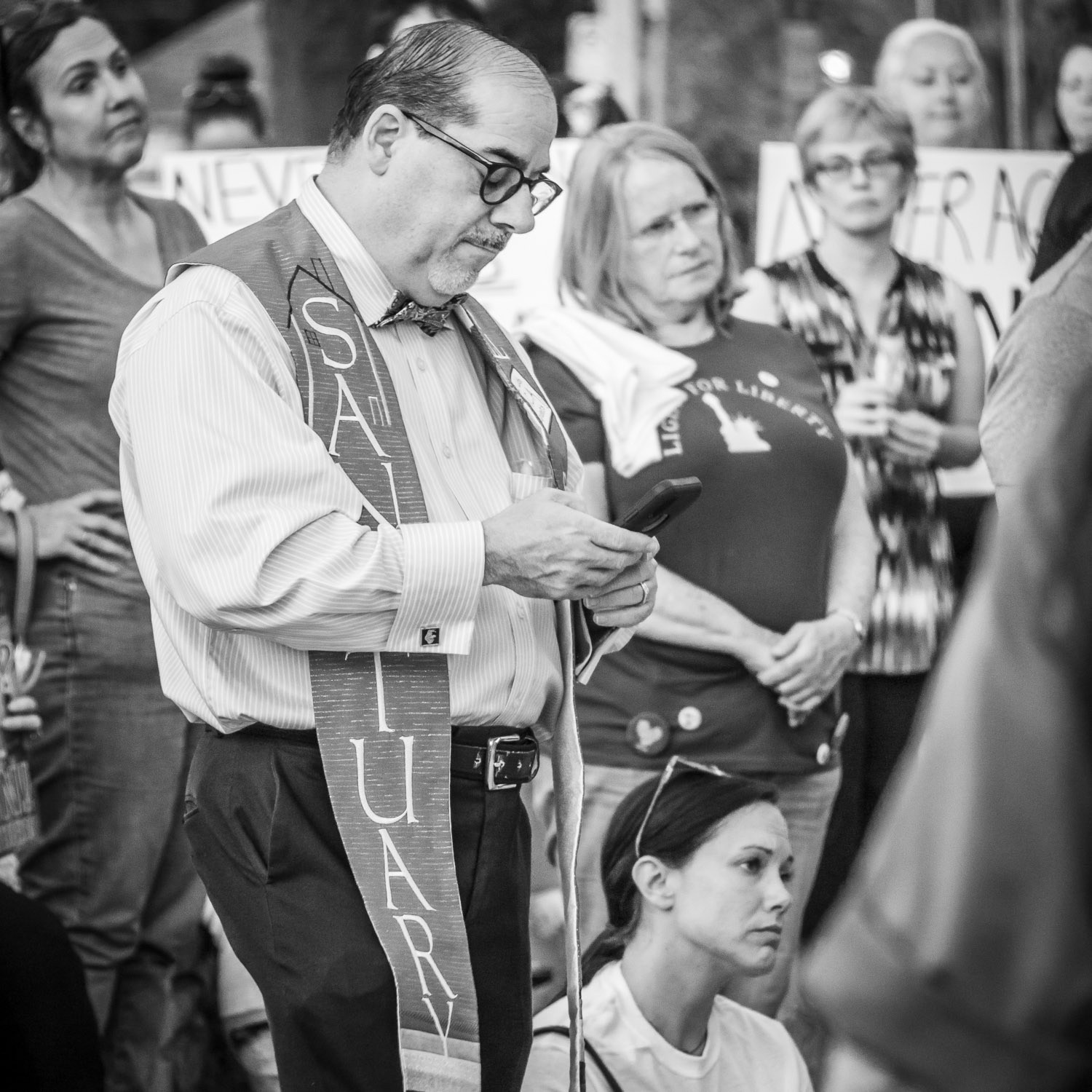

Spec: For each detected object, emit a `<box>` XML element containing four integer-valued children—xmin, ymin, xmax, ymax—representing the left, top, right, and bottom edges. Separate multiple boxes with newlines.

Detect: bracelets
<box><xmin>826</xmin><ymin>604</ymin><xmax>866</xmax><ymax>643</ymax></box>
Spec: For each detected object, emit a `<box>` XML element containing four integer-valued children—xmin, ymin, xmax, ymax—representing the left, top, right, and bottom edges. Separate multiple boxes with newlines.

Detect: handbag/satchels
<box><xmin>0</xmin><ymin>504</ymin><xmax>52</xmax><ymax>859</ymax></box>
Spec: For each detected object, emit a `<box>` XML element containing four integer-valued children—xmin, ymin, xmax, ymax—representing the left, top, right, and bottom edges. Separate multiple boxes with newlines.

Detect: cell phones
<box><xmin>612</xmin><ymin>477</ymin><xmax>704</xmax><ymax>538</ymax></box>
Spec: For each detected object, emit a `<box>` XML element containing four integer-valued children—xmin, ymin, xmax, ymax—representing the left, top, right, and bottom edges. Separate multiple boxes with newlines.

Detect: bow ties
<box><xmin>368</xmin><ymin>290</ymin><xmax>470</xmax><ymax>338</ymax></box>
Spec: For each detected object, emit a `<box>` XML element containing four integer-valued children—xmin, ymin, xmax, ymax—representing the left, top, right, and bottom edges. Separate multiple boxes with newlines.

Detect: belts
<box><xmin>202</xmin><ymin>721</ymin><xmax>542</xmax><ymax>793</ymax></box>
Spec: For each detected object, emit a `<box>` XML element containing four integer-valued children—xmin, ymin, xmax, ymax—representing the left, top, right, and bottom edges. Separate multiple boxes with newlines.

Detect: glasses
<box><xmin>395</xmin><ymin>107</ymin><xmax>565</xmax><ymax>219</ymax></box>
<box><xmin>633</xmin><ymin>753</ymin><xmax>736</xmax><ymax>862</ymax></box>
<box><xmin>802</xmin><ymin>149</ymin><xmax>906</xmax><ymax>181</ymax></box>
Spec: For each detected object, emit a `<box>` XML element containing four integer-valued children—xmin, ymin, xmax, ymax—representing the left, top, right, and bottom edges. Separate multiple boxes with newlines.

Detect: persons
<box><xmin>795</xmin><ymin>16</ymin><xmax>1092</xmax><ymax>1092</ymax></box>
<box><xmin>0</xmin><ymin>0</ymin><xmax>220</xmax><ymax>1092</ymax></box>
<box><xmin>519</xmin><ymin>770</ymin><xmax>816</xmax><ymax>1092</ymax></box>
<box><xmin>512</xmin><ymin>119</ymin><xmax>881</xmax><ymax>1019</ymax></box>
<box><xmin>177</xmin><ymin>55</ymin><xmax>265</xmax><ymax>152</ymax></box>
<box><xmin>727</xmin><ymin>84</ymin><xmax>987</xmax><ymax>949</ymax></box>
<box><xmin>105</xmin><ymin>18</ymin><xmax>661</xmax><ymax>1091</ymax></box>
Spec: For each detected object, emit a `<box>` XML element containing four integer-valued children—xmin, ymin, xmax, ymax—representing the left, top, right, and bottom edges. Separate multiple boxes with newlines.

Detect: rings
<box><xmin>634</xmin><ymin>582</ymin><xmax>649</xmax><ymax>606</ymax></box>
